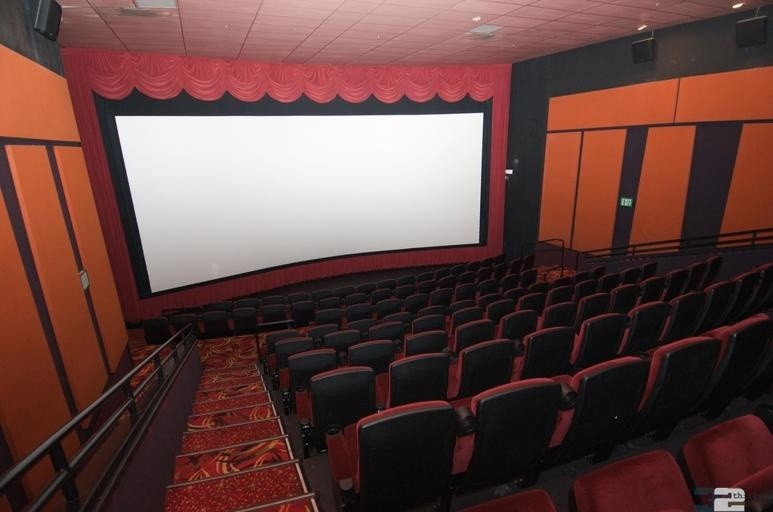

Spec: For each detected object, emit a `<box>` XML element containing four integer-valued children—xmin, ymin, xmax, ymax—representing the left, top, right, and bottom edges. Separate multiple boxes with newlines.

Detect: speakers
<box><xmin>33</xmin><ymin>0</ymin><xmax>62</xmax><ymax>41</ymax></box>
<box><xmin>736</xmin><ymin>16</ymin><xmax>766</xmax><ymax>47</ymax></box>
<box><xmin>631</xmin><ymin>38</ymin><xmax>655</xmax><ymax>63</ymax></box>
